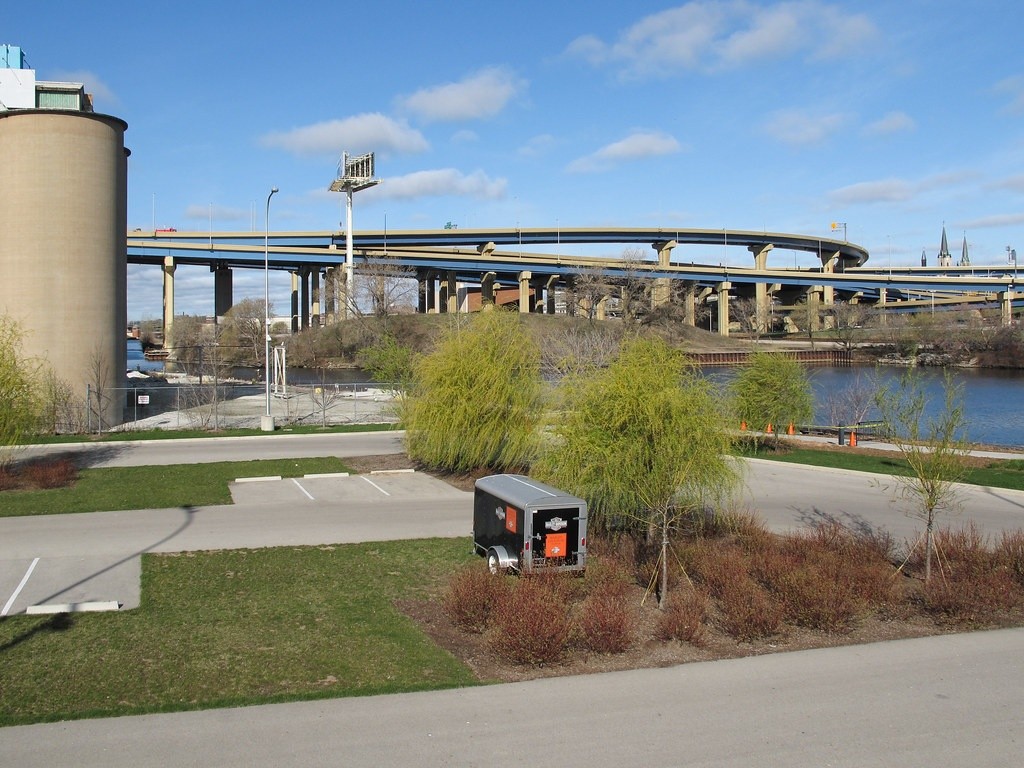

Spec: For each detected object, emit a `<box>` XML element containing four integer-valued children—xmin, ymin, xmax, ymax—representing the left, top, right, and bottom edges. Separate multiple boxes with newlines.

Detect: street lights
<box><xmin>770</xmin><ymin>301</ymin><xmax>775</xmax><ymax>333</ymax></box>
<box><xmin>887</xmin><ymin>234</ymin><xmax>891</xmax><ymax>276</ymax></box>
<box><xmin>555</xmin><ymin>219</ymin><xmax>560</xmax><ymax>260</ymax></box>
<box><xmin>722</xmin><ymin>227</ymin><xmax>728</xmax><ymax>269</ymax></box>
<box><xmin>383</xmin><ymin>209</ymin><xmax>388</xmax><ymax>256</ymax></box>
<box><xmin>930</xmin><ymin>290</ymin><xmax>936</xmax><ymax>323</ymax></box>
<box><xmin>708</xmin><ymin>305</ymin><xmax>713</xmax><ymax>332</ymax></box>
<box><xmin>265</xmin><ymin>187</ymin><xmax>279</xmax><ymax>417</ymax></box>
<box><xmin>516</xmin><ymin>221</ymin><xmax>522</xmax><ymax>258</ymax></box>
<box><xmin>882</xmin><ymin>291</ymin><xmax>888</xmax><ymax>305</ymax></box>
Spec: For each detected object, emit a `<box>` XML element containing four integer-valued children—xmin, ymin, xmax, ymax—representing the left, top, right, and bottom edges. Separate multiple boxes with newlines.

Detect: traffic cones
<box><xmin>740</xmin><ymin>421</ymin><xmax>747</xmax><ymax>431</ymax></box>
<box><xmin>848</xmin><ymin>431</ymin><xmax>856</xmax><ymax>447</ymax></box>
<box><xmin>766</xmin><ymin>422</ymin><xmax>773</xmax><ymax>434</ymax></box>
<box><xmin>787</xmin><ymin>422</ymin><xmax>795</xmax><ymax>435</ymax></box>
